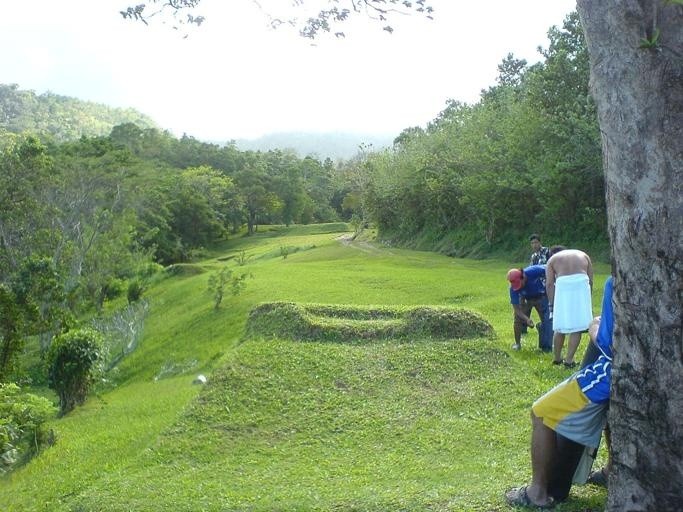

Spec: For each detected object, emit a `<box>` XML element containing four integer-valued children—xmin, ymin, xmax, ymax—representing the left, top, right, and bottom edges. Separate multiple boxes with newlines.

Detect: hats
<box><xmin>507</xmin><ymin>269</ymin><xmax>522</xmax><ymax>291</ymax></box>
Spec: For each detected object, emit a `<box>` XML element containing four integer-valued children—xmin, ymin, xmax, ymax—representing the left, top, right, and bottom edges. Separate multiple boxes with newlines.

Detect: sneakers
<box><xmin>562</xmin><ymin>360</ymin><xmax>580</xmax><ymax>368</ymax></box>
<box><xmin>552</xmin><ymin>360</ymin><xmax>563</xmax><ymax>365</ymax></box>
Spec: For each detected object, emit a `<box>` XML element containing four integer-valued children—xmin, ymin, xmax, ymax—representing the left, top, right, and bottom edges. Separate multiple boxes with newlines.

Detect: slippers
<box><xmin>587</xmin><ymin>467</ymin><xmax>610</xmax><ymax>489</ymax></box>
<box><xmin>506</xmin><ymin>485</ymin><xmax>555</xmax><ymax>509</ymax></box>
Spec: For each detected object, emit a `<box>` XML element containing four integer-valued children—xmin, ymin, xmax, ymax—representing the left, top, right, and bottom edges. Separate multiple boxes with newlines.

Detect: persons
<box><xmin>502</xmin><ymin>267</ymin><xmax>615</xmax><ymax>510</ymax></box>
<box><xmin>506</xmin><ymin>265</ymin><xmax>548</xmax><ymax>350</ymax></box>
<box><xmin>527</xmin><ymin>234</ymin><xmax>550</xmax><ymax>267</ymax></box>
<box><xmin>544</xmin><ymin>245</ymin><xmax>594</xmax><ymax>368</ymax></box>
